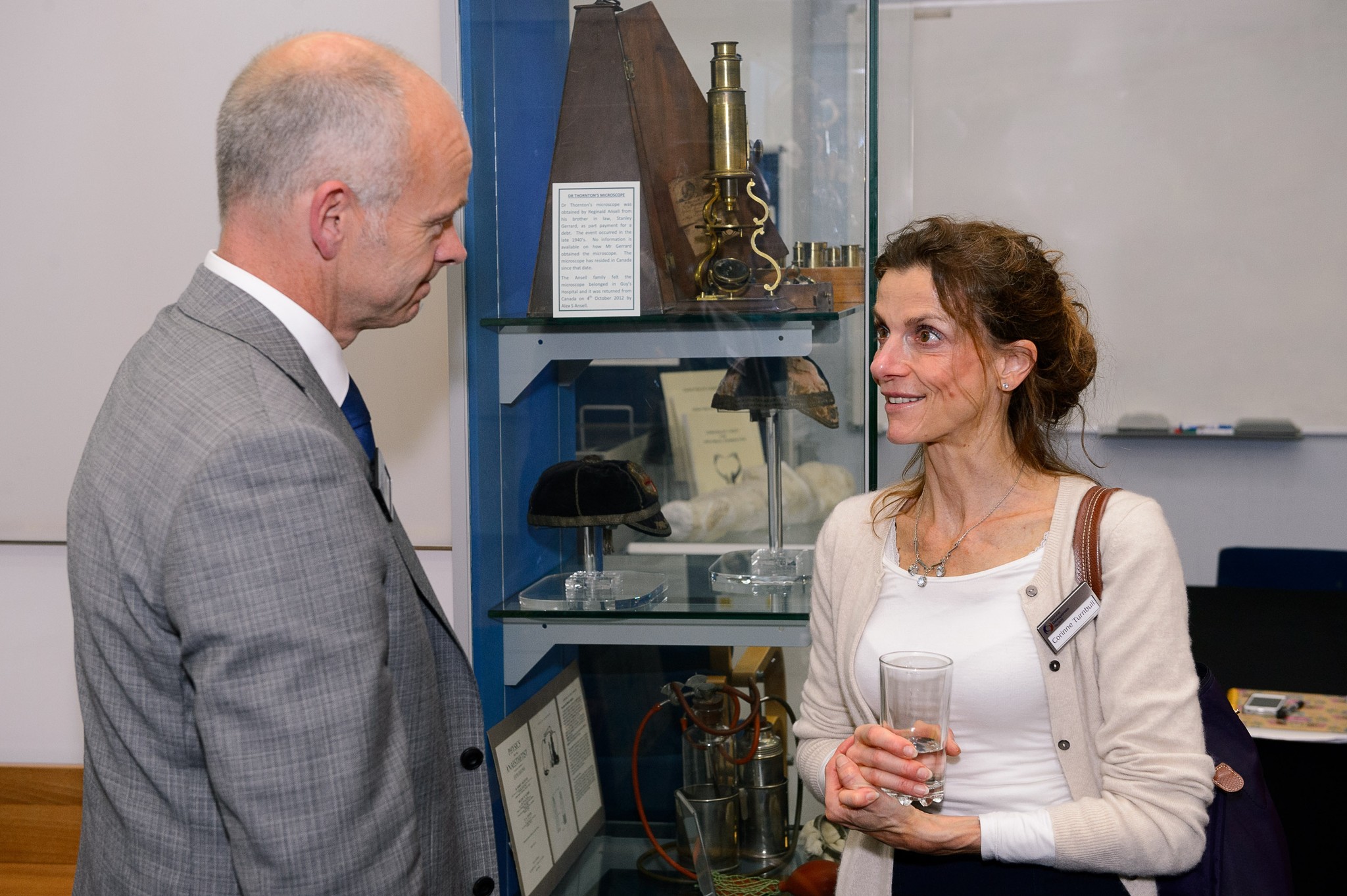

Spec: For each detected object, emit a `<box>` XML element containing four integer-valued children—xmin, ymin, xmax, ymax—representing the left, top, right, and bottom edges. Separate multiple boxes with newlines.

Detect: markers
<box><xmin>1176</xmin><ymin>423</ymin><xmax>1234</xmax><ymax>436</ymax></box>
<box><xmin>1276</xmin><ymin>700</ymin><xmax>1305</xmax><ymax>719</ymax></box>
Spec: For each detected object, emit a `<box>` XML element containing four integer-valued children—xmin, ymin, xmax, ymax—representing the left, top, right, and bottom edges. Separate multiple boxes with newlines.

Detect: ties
<box><xmin>344</xmin><ymin>374</ymin><xmax>374</xmax><ymax>470</ymax></box>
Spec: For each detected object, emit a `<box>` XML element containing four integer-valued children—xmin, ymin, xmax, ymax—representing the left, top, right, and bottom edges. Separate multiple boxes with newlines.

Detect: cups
<box><xmin>879</xmin><ymin>652</ymin><xmax>953</xmax><ymax>805</ymax></box>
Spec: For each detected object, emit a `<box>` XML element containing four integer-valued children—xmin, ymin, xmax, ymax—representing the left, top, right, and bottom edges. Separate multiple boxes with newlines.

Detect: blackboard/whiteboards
<box><xmin>866</xmin><ymin>1</ymin><xmax>1347</xmax><ymax>438</ymax></box>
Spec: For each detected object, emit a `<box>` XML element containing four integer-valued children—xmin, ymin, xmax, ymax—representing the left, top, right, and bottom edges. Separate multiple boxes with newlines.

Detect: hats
<box><xmin>713</xmin><ymin>354</ymin><xmax>840</xmax><ymax>429</ymax></box>
<box><xmin>528</xmin><ymin>454</ymin><xmax>672</xmax><ymax>536</ymax></box>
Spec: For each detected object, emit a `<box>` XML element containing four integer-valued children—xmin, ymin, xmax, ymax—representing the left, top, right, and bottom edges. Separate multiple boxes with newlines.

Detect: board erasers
<box><xmin>1233</xmin><ymin>418</ymin><xmax>1303</xmax><ymax>436</ymax></box>
<box><xmin>1117</xmin><ymin>413</ymin><xmax>1170</xmax><ymax>435</ymax></box>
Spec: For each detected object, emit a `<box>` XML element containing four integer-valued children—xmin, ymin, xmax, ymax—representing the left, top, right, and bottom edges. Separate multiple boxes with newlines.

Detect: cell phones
<box><xmin>1243</xmin><ymin>692</ymin><xmax>1286</xmax><ymax>716</ymax></box>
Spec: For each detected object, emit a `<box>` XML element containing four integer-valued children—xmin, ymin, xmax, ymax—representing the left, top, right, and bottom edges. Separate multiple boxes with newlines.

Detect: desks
<box><xmin>1228</xmin><ymin>688</ymin><xmax>1347</xmax><ymax>743</ymax></box>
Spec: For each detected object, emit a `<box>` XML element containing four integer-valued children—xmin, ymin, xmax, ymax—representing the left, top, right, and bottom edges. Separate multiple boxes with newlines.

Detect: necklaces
<box><xmin>904</xmin><ymin>453</ymin><xmax>1026</xmax><ymax>589</ymax></box>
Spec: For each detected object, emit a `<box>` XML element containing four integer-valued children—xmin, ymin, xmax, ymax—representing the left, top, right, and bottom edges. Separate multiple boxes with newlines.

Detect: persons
<box><xmin>66</xmin><ymin>32</ymin><xmax>500</xmax><ymax>896</ymax></box>
<box><xmin>790</xmin><ymin>217</ymin><xmax>1215</xmax><ymax>896</ymax></box>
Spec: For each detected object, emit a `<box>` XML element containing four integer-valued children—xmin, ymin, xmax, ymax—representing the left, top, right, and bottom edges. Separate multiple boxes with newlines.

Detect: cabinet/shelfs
<box><xmin>454</xmin><ymin>0</ymin><xmax>879</xmax><ymax>896</ymax></box>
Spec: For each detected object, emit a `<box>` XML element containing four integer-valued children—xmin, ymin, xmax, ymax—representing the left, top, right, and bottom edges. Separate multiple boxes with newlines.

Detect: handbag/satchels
<box><xmin>1077</xmin><ymin>483</ymin><xmax>1289</xmax><ymax>895</ymax></box>
